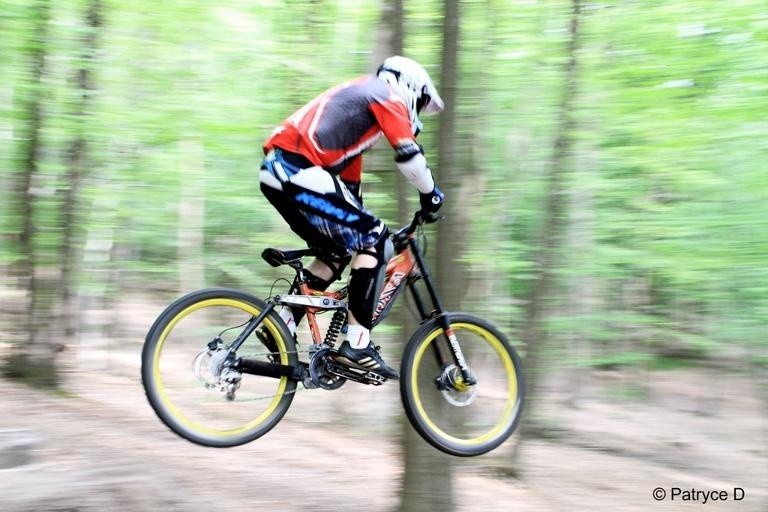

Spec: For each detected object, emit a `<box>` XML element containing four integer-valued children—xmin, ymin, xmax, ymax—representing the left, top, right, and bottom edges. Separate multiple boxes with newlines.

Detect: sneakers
<box><xmin>337</xmin><ymin>340</ymin><xmax>402</xmax><ymax>379</ymax></box>
<box><xmin>256</xmin><ymin>324</ymin><xmax>297</xmax><ymax>363</ymax></box>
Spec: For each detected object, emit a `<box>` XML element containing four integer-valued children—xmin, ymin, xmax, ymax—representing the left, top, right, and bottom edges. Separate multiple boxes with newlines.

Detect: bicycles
<box><xmin>140</xmin><ymin>195</ymin><xmax>526</xmax><ymax>457</ymax></box>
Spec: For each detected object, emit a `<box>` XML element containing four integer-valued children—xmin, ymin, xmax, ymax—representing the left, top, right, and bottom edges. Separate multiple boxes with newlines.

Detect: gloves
<box><xmin>418</xmin><ymin>183</ymin><xmax>445</xmax><ymax>212</ymax></box>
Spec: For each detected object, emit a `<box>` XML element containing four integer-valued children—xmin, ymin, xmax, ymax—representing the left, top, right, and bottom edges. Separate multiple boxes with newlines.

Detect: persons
<box><xmin>259</xmin><ymin>55</ymin><xmax>449</xmax><ymax>380</ymax></box>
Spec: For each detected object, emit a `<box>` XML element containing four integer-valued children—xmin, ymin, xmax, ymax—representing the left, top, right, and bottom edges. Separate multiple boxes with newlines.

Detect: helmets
<box><xmin>377</xmin><ymin>55</ymin><xmax>445</xmax><ymax>113</ymax></box>
